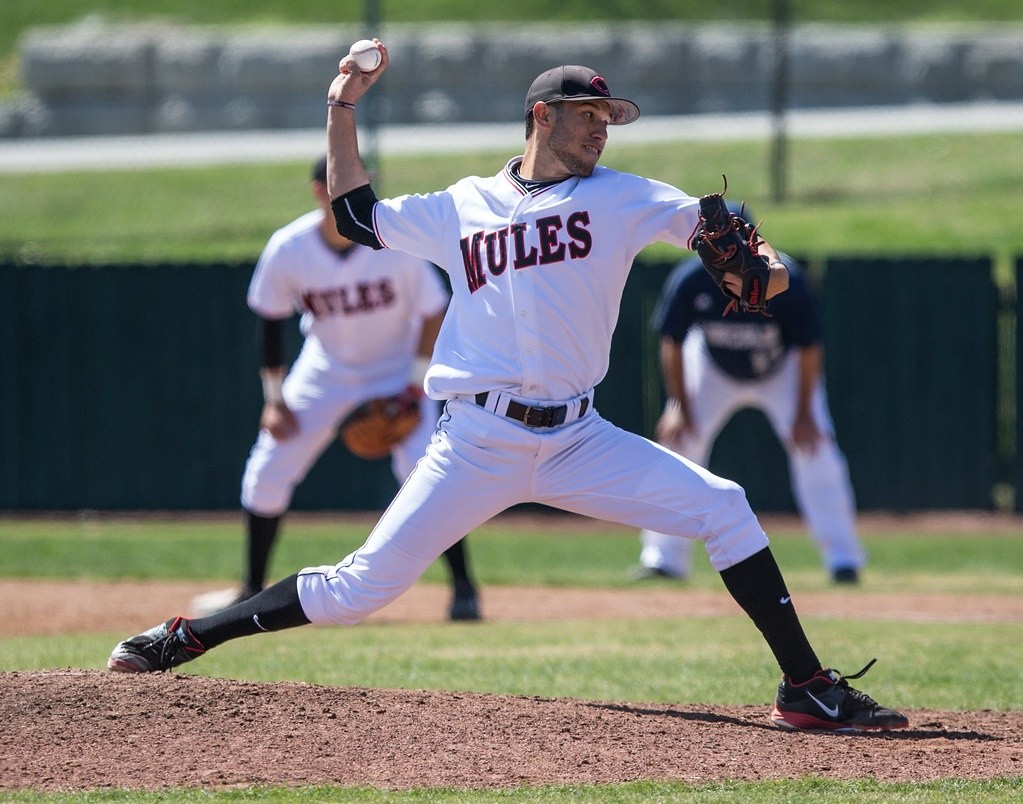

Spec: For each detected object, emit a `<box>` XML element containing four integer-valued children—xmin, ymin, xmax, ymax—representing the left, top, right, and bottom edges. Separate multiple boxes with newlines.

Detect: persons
<box><xmin>219</xmin><ymin>153</ymin><xmax>478</xmax><ymax>620</ymax></box>
<box><xmin>626</xmin><ymin>199</ymin><xmax>866</xmax><ymax>582</ymax></box>
<box><xmin>108</xmin><ymin>37</ymin><xmax>909</xmax><ymax>732</ymax></box>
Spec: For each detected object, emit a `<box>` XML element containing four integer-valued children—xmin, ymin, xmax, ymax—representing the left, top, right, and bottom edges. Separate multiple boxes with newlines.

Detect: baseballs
<box><xmin>349</xmin><ymin>39</ymin><xmax>382</xmax><ymax>73</ymax></box>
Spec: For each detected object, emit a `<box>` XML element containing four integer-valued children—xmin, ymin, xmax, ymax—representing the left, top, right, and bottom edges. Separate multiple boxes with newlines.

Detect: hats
<box><xmin>314</xmin><ymin>157</ymin><xmax>326</xmax><ymax>180</ymax></box>
<box><xmin>729</xmin><ymin>203</ymin><xmax>751</xmax><ymax>226</ymax></box>
<box><xmin>524</xmin><ymin>65</ymin><xmax>640</xmax><ymax>125</ymax></box>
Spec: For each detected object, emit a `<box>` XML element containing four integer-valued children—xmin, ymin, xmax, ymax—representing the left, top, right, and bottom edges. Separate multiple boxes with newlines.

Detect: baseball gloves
<box><xmin>343</xmin><ymin>386</ymin><xmax>422</xmax><ymax>460</ymax></box>
<box><xmin>691</xmin><ymin>192</ymin><xmax>772</xmax><ymax>311</ymax></box>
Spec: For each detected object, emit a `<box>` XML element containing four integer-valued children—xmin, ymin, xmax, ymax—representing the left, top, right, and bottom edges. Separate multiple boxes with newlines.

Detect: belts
<box><xmin>475</xmin><ymin>392</ymin><xmax>589</xmax><ymax>427</ymax></box>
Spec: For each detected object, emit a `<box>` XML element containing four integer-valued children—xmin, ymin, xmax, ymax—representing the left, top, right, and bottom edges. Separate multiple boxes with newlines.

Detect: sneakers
<box><xmin>771</xmin><ymin>658</ymin><xmax>909</xmax><ymax>731</ymax></box>
<box><xmin>108</xmin><ymin>617</ymin><xmax>206</xmax><ymax>672</ymax></box>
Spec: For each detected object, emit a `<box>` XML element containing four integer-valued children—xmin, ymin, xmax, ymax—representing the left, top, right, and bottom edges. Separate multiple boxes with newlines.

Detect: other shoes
<box><xmin>634</xmin><ymin>567</ymin><xmax>674</xmax><ymax>580</ymax></box>
<box><xmin>833</xmin><ymin>568</ymin><xmax>859</xmax><ymax>584</ymax></box>
<box><xmin>453</xmin><ymin>585</ymin><xmax>478</xmax><ymax>620</ymax></box>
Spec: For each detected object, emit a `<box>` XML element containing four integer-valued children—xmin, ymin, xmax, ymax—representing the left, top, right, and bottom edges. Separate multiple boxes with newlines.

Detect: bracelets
<box><xmin>326</xmin><ymin>100</ymin><xmax>357</xmax><ymax>112</ymax></box>
